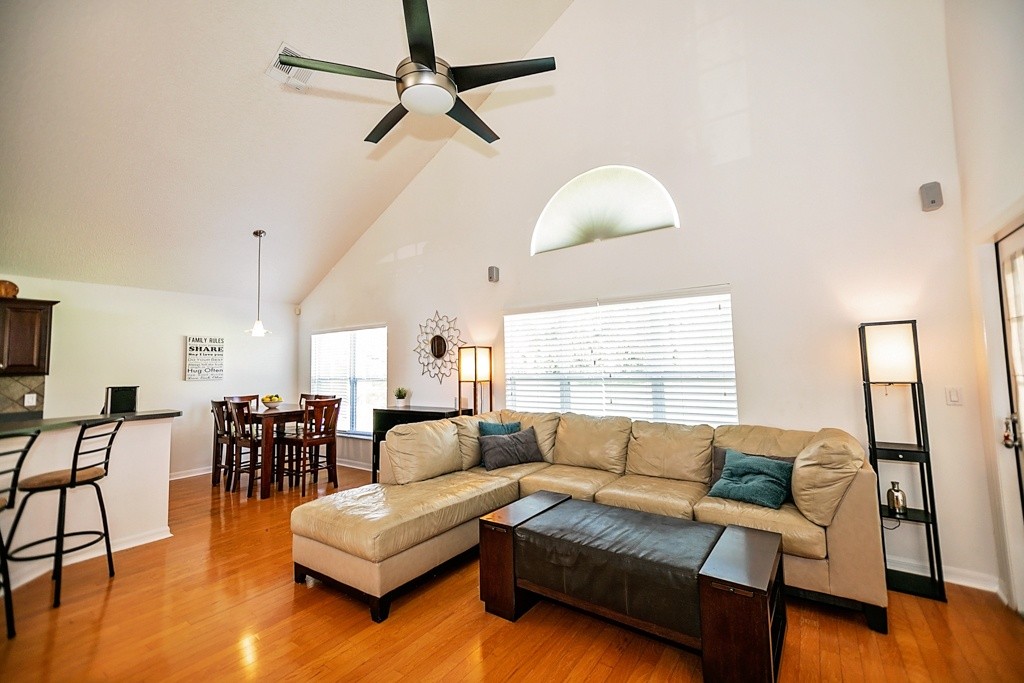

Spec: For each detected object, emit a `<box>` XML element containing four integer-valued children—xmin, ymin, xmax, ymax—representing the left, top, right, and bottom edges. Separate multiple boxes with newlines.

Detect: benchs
<box><xmin>478</xmin><ymin>489</ymin><xmax>789</xmax><ymax>683</ymax></box>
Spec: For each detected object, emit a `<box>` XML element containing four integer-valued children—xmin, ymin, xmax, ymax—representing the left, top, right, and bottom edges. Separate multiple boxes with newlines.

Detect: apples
<box><xmin>262</xmin><ymin>395</ymin><xmax>282</xmax><ymax>401</ymax></box>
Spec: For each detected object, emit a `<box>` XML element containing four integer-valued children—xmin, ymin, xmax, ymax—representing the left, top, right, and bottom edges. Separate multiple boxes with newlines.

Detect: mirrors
<box><xmin>413</xmin><ymin>309</ymin><xmax>467</xmax><ymax>384</ymax></box>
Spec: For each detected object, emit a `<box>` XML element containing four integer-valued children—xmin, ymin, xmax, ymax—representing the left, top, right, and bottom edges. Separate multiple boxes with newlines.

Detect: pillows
<box><xmin>477</xmin><ymin>426</ymin><xmax>544</xmax><ymax>472</ymax></box>
<box><xmin>478</xmin><ymin>421</ymin><xmax>520</xmax><ymax>467</ymax></box>
<box><xmin>711</xmin><ymin>445</ymin><xmax>796</xmax><ymax>487</ymax></box>
<box><xmin>707</xmin><ymin>448</ymin><xmax>793</xmax><ymax>510</ymax></box>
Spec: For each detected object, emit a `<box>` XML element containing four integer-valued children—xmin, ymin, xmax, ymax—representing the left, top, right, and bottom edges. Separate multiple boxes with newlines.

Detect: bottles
<box><xmin>887</xmin><ymin>481</ymin><xmax>907</xmax><ymax>520</ymax></box>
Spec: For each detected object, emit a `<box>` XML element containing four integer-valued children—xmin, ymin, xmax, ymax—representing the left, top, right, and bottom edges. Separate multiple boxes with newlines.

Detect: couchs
<box><xmin>290</xmin><ymin>410</ymin><xmax>889</xmax><ymax>638</ymax></box>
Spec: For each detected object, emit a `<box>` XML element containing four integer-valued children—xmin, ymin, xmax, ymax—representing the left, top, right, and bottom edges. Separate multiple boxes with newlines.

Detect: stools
<box><xmin>0</xmin><ymin>429</ymin><xmax>41</xmax><ymax>639</ymax></box>
<box><xmin>0</xmin><ymin>414</ymin><xmax>126</xmax><ymax>608</ymax></box>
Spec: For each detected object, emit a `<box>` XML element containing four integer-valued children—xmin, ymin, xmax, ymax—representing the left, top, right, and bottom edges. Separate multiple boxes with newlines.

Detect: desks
<box><xmin>211</xmin><ymin>404</ymin><xmax>338</xmax><ymax>499</ymax></box>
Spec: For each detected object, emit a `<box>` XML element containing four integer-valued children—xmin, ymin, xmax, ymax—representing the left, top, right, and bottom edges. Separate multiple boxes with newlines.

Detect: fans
<box><xmin>279</xmin><ymin>0</ymin><xmax>556</xmax><ymax>143</ymax></box>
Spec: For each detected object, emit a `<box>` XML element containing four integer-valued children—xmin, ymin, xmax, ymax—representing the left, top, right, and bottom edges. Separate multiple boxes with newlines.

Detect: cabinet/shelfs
<box><xmin>858</xmin><ymin>319</ymin><xmax>948</xmax><ymax>604</ymax></box>
<box><xmin>372</xmin><ymin>405</ymin><xmax>473</xmax><ymax>484</ymax></box>
<box><xmin>0</xmin><ymin>295</ymin><xmax>61</xmax><ymax>376</ymax></box>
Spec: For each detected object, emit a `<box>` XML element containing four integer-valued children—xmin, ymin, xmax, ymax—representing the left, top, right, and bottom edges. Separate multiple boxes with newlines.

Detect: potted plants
<box><xmin>392</xmin><ymin>385</ymin><xmax>407</xmax><ymax>406</ymax></box>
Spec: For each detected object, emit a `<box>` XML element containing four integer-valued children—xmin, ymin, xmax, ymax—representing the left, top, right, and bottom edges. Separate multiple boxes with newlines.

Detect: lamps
<box><xmin>396</xmin><ymin>72</ymin><xmax>459</xmax><ymax>117</ymax></box>
<box><xmin>458</xmin><ymin>346</ymin><xmax>492</xmax><ymax>416</ymax></box>
<box><xmin>251</xmin><ymin>229</ymin><xmax>268</xmax><ymax>337</ymax></box>
<box><xmin>864</xmin><ymin>325</ymin><xmax>918</xmax><ymax>397</ymax></box>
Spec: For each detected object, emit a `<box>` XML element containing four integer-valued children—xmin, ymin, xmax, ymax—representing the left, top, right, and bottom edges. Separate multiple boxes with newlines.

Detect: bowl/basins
<box><xmin>263</xmin><ymin>401</ymin><xmax>283</xmax><ymax>409</ymax></box>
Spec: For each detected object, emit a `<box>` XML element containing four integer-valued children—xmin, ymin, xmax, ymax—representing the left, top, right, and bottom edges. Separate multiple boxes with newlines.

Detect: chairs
<box><xmin>211</xmin><ymin>394</ymin><xmax>342</xmax><ymax>498</ymax></box>
